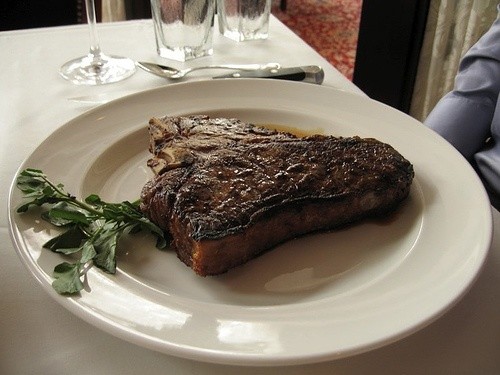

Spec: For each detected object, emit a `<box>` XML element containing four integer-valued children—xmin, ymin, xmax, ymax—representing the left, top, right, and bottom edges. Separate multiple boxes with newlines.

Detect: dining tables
<box><xmin>0</xmin><ymin>13</ymin><xmax>499</xmax><ymax>375</ymax></box>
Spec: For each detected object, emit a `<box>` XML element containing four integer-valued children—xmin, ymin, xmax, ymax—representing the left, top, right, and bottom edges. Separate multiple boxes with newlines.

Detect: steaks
<box><xmin>147</xmin><ymin>114</ymin><xmax>298</xmax><ymax>165</ymax></box>
<box><xmin>137</xmin><ymin>134</ymin><xmax>416</xmax><ymax>276</ymax></box>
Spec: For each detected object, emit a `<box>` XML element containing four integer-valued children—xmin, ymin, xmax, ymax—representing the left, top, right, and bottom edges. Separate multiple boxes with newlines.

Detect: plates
<box><xmin>7</xmin><ymin>77</ymin><xmax>495</xmax><ymax>368</ymax></box>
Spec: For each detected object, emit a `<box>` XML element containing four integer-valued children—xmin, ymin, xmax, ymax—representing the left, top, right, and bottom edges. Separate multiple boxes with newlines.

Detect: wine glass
<box><xmin>60</xmin><ymin>0</ymin><xmax>135</xmax><ymax>86</ymax></box>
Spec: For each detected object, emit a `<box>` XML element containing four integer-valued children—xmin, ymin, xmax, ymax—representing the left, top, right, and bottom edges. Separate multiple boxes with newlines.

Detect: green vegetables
<box><xmin>13</xmin><ymin>168</ymin><xmax>173</xmax><ymax>294</ymax></box>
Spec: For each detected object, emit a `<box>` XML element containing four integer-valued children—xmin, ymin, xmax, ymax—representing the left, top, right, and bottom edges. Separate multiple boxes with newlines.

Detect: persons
<box><xmin>424</xmin><ymin>3</ymin><xmax>500</xmax><ymax>212</ymax></box>
<box><xmin>0</xmin><ymin>0</ymin><xmax>182</xmax><ymax>32</ymax></box>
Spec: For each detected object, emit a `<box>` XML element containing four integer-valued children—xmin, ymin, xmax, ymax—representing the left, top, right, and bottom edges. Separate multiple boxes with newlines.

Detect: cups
<box><xmin>150</xmin><ymin>0</ymin><xmax>216</xmax><ymax>64</ymax></box>
<box><xmin>216</xmin><ymin>0</ymin><xmax>271</xmax><ymax>42</ymax></box>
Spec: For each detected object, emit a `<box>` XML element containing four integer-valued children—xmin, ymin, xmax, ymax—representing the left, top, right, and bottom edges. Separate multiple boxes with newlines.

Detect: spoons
<box><xmin>134</xmin><ymin>59</ymin><xmax>280</xmax><ymax>80</ymax></box>
